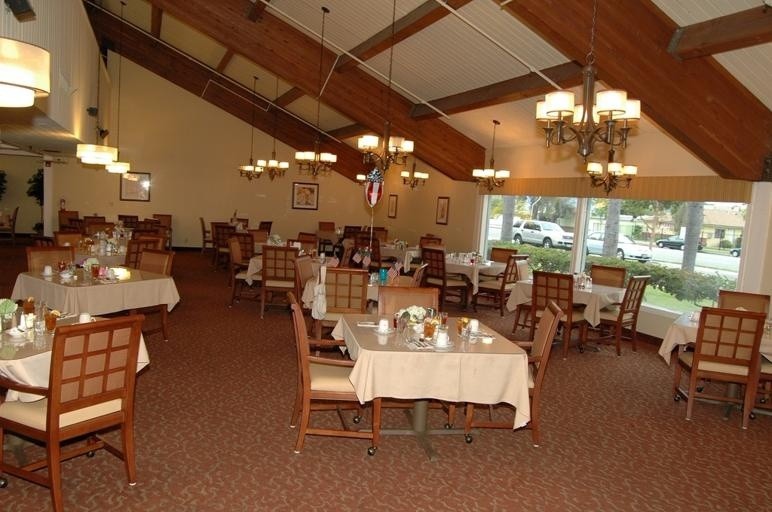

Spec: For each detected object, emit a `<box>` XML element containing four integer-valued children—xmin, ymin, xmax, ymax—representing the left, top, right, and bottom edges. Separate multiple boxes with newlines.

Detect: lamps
<box><xmin>472</xmin><ymin>120</ymin><xmax>510</xmax><ymax>193</ymax></box>
<box><xmin>0</xmin><ymin>0</ymin><xmax>130</xmax><ymax>174</ymax></box>
<box><xmin>536</xmin><ymin>0</ymin><xmax>641</xmax><ymax>197</ymax></box>
<box><xmin>238</xmin><ymin>0</ymin><xmax>430</xmax><ymax>191</ymax></box>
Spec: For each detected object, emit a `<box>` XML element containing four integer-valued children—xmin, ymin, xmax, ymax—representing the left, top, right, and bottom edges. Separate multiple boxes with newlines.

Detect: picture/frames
<box><xmin>388</xmin><ymin>194</ymin><xmax>398</xmax><ymax>218</ymax></box>
<box><xmin>292</xmin><ymin>182</ymin><xmax>319</xmax><ymax>210</ymax></box>
<box><xmin>120</xmin><ymin>171</ymin><xmax>151</xmax><ymax>202</ymax></box>
<box><xmin>436</xmin><ymin>196</ymin><xmax>450</xmax><ymax>225</ymax></box>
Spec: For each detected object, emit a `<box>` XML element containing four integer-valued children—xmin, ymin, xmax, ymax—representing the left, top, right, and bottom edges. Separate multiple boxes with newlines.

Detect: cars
<box><xmin>730</xmin><ymin>247</ymin><xmax>742</xmax><ymax>257</ymax></box>
<box><xmin>584</xmin><ymin>232</ymin><xmax>653</xmax><ymax>264</ymax></box>
<box><xmin>657</xmin><ymin>234</ymin><xmax>704</xmax><ymax>252</ymax></box>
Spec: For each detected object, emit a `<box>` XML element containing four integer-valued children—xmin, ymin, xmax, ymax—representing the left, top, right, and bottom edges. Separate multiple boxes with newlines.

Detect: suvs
<box><xmin>510</xmin><ymin>218</ymin><xmax>574</xmax><ymax>252</ymax></box>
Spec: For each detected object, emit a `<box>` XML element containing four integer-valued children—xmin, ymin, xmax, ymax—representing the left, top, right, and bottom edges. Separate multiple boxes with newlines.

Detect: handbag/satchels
<box><xmin>311</xmin><ymin>265</ymin><xmax>327</xmax><ymax>321</ymax></box>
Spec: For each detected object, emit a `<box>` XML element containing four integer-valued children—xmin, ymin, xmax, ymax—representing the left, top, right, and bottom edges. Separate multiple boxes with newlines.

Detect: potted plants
<box><xmin>26</xmin><ymin>168</ymin><xmax>44</xmax><ymax>235</ymax></box>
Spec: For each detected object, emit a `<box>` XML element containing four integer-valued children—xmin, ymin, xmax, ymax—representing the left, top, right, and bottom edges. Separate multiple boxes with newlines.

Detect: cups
<box><xmin>77</xmin><ymin>311</ymin><xmax>98</xmax><ymax>327</ymax></box>
<box><xmin>320</xmin><ymin>253</ymin><xmax>326</xmax><ymax>259</ymax></box>
<box><xmin>100</xmin><ymin>229</ymin><xmax>121</xmax><ymax>252</ymax></box>
<box><xmin>44</xmin><ymin>260</ymin><xmax>116</xmax><ymax>281</ymax></box>
<box><xmin>19</xmin><ymin>297</ymin><xmax>57</xmax><ymax>336</ymax></box>
<box><xmin>463</xmin><ymin>252</ymin><xmax>482</xmax><ymax>265</ymax></box>
<box><xmin>573</xmin><ymin>270</ymin><xmax>593</xmax><ymax>290</ymax></box>
<box><xmin>380</xmin><ymin>268</ymin><xmax>387</xmax><ymax>281</ymax></box>
<box><xmin>377</xmin><ymin>312</ymin><xmax>493</xmax><ymax>348</ymax></box>
